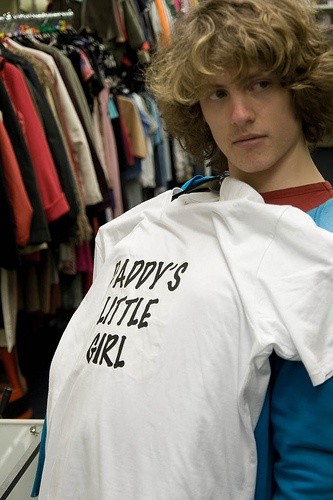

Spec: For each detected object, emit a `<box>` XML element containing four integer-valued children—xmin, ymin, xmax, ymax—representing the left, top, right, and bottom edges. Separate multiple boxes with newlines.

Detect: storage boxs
<box><xmin>0</xmin><ymin>419</ymin><xmax>45</xmax><ymax>500</ymax></box>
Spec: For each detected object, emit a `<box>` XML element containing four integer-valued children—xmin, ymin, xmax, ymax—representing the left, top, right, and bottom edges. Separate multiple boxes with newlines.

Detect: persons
<box><xmin>31</xmin><ymin>0</ymin><xmax>333</xmax><ymax>500</ymax></box>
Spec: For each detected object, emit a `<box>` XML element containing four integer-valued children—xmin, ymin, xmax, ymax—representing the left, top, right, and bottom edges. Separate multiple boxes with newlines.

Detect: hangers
<box><xmin>0</xmin><ymin>12</ymin><xmax>79</xmax><ymax>41</ymax></box>
